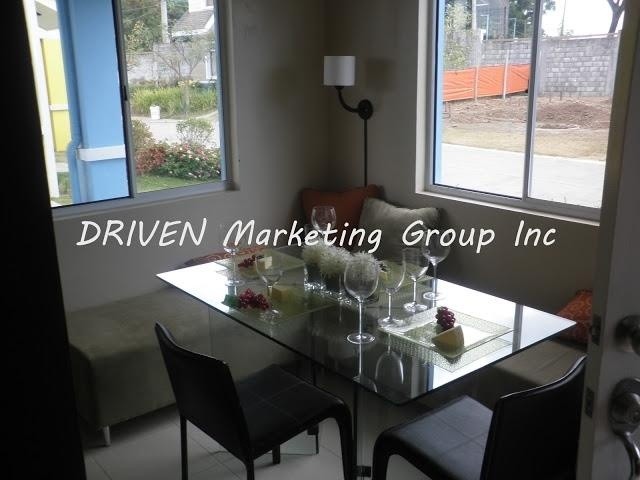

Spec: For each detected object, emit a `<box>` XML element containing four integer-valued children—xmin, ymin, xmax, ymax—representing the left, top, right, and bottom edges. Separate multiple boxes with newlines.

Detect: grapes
<box><xmin>238</xmin><ymin>255</ymin><xmax>262</xmax><ymax>267</ymax></box>
<box><xmin>435</xmin><ymin>307</ymin><xmax>454</xmax><ymax>330</ymax></box>
<box><xmin>238</xmin><ymin>289</ymin><xmax>269</xmax><ymax>309</ymax></box>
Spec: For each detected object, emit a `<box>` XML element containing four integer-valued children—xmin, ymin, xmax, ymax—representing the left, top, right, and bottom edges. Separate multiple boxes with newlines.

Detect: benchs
<box><xmin>65</xmin><ymin>248</ymin><xmax>311</xmax><ymax>446</ymax></box>
<box><xmin>312</xmin><ymin>265</ymin><xmax>587</xmax><ymax>411</ymax></box>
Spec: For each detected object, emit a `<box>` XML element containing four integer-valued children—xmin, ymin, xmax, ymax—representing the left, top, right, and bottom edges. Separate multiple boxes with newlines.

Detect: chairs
<box><xmin>373</xmin><ymin>356</ymin><xmax>586</xmax><ymax>480</ymax></box>
<box><xmin>155</xmin><ymin>322</ymin><xmax>356</xmax><ymax>480</ymax></box>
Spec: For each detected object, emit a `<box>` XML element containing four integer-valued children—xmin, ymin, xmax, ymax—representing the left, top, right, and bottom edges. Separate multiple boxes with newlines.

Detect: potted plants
<box><xmin>300</xmin><ymin>237</ymin><xmax>392</xmax><ymax>308</ymax></box>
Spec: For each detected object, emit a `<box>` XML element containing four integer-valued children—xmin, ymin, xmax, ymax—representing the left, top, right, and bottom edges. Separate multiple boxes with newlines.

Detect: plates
<box><xmin>377</xmin><ymin>305</ymin><xmax>516</xmax><ymax>359</ymax></box>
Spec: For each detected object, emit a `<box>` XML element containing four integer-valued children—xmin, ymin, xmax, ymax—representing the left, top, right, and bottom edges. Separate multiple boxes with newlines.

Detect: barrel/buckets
<box><xmin>150</xmin><ymin>105</ymin><xmax>161</xmax><ymax>120</ymax></box>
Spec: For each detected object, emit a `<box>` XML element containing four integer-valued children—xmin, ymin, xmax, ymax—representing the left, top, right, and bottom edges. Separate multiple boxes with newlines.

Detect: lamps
<box><xmin>324</xmin><ymin>55</ymin><xmax>373</xmax><ymax>187</ymax></box>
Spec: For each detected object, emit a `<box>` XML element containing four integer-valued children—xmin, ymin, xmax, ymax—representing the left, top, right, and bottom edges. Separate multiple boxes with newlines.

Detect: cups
<box><xmin>303</xmin><ymin>263</ymin><xmax>378</xmax><ymax>305</ymax></box>
<box><xmin>305</xmin><ymin>223</ymin><xmax>327</xmax><ymax>243</ymax></box>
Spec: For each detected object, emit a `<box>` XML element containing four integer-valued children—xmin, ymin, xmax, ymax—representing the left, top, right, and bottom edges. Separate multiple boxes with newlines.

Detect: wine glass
<box><xmin>422</xmin><ymin>231</ymin><xmax>451</xmax><ymax>301</ymax></box>
<box><xmin>376</xmin><ymin>261</ymin><xmax>405</xmax><ymax>328</ymax></box>
<box><xmin>345</xmin><ymin>259</ymin><xmax>379</xmax><ymax>344</ymax></box>
<box><xmin>312</xmin><ymin>206</ymin><xmax>337</xmax><ymax>240</ymax></box>
<box><xmin>255</xmin><ymin>256</ymin><xmax>283</xmax><ymax>319</ymax></box>
<box><xmin>218</xmin><ymin>219</ymin><xmax>246</xmax><ymax>288</ymax></box>
<box><xmin>402</xmin><ymin>248</ymin><xmax>430</xmax><ymax>313</ymax></box>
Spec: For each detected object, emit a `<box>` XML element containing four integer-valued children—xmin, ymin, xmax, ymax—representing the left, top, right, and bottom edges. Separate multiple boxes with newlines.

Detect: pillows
<box><xmin>555</xmin><ymin>289</ymin><xmax>592</xmax><ymax>344</ymax></box>
<box><xmin>184</xmin><ymin>243</ymin><xmax>268</xmax><ymax>266</ymax></box>
<box><xmin>297</xmin><ymin>184</ymin><xmax>444</xmax><ymax>275</ymax></box>
